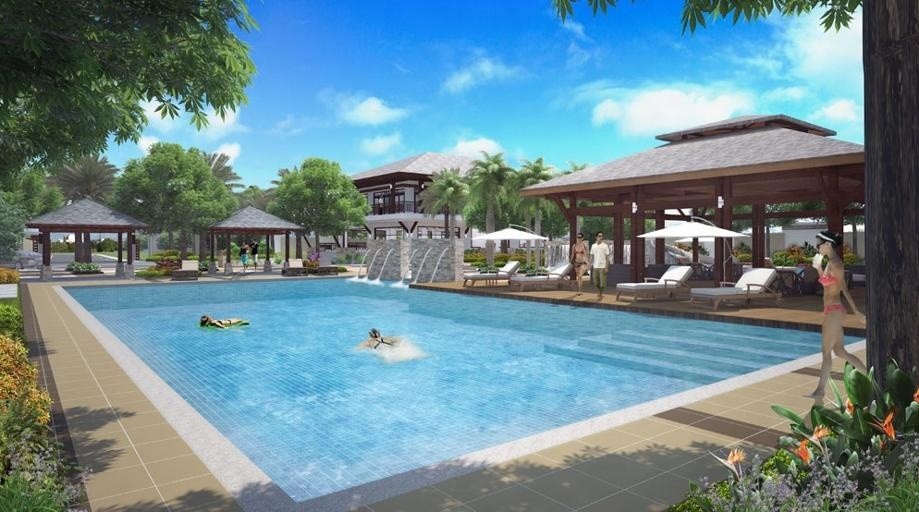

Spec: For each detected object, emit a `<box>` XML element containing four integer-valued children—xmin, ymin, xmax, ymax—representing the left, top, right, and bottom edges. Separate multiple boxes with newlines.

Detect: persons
<box><xmin>800</xmin><ymin>231</ymin><xmax>866</xmax><ymax>399</ymax></box>
<box><xmin>354</xmin><ymin>327</ymin><xmax>400</xmax><ymax>351</ymax></box>
<box><xmin>587</xmin><ymin>231</ymin><xmax>610</xmax><ymax>299</ymax></box>
<box><xmin>199</xmin><ymin>314</ymin><xmax>247</xmax><ymax>329</ymax></box>
<box><xmin>569</xmin><ymin>234</ymin><xmax>588</xmax><ymax>296</ymax></box>
<box><xmin>239</xmin><ymin>240</ymin><xmax>250</xmax><ymax>270</ymax></box>
<box><xmin>249</xmin><ymin>239</ymin><xmax>260</xmax><ymax>271</ymax></box>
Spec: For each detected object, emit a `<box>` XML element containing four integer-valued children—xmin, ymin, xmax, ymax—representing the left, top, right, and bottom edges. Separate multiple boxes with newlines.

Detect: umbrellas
<box><xmin>471</xmin><ymin>224</ymin><xmax>551</xmax><ymax>275</ymax></box>
<box><xmin>636</xmin><ymin>214</ymin><xmax>749</xmax><ymax>289</ymax></box>
<box><xmin>676</xmin><ymin>239</ymin><xmax>715</xmax><ymax>242</ymax></box>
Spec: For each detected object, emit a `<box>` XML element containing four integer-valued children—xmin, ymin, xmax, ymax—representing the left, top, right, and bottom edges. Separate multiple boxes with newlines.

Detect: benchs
<box><xmin>173</xmin><ymin>268</ymin><xmax>199</xmax><ymax>280</ymax></box>
<box><xmin>317</xmin><ymin>266</ymin><xmax>339</xmax><ymax>276</ymax></box>
<box><xmin>283</xmin><ymin>267</ymin><xmax>310</xmax><ymax>277</ymax></box>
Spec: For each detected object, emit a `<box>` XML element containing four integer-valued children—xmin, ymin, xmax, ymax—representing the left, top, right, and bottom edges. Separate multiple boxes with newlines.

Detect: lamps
<box><xmin>718</xmin><ymin>196</ymin><xmax>724</xmax><ymax>208</ymax></box>
<box><xmin>632</xmin><ymin>202</ymin><xmax>638</xmax><ymax>214</ymax></box>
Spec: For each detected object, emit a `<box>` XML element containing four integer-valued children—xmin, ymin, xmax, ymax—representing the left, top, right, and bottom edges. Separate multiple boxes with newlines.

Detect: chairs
<box><xmin>461</xmin><ymin>259</ymin><xmax>573</xmax><ymax>291</ymax></box>
<box><xmin>616</xmin><ymin>265</ymin><xmax>783</xmax><ymax>311</ymax></box>
